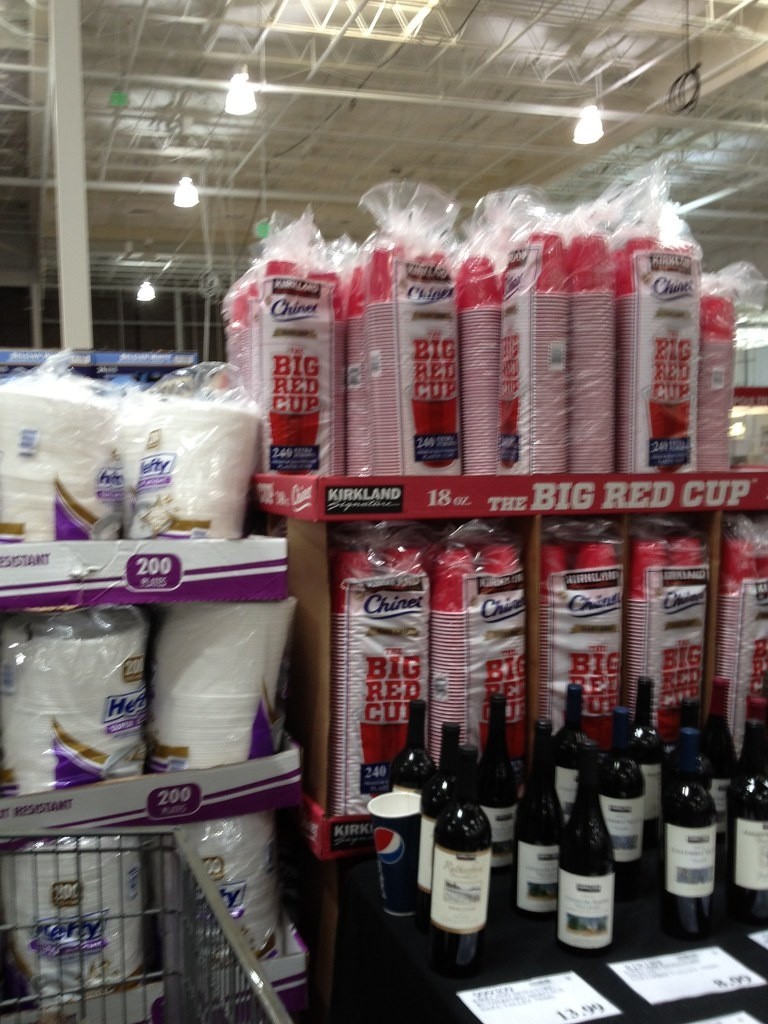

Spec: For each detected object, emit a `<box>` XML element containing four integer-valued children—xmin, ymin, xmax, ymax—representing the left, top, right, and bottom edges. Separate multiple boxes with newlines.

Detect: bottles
<box><xmin>660</xmin><ymin>728</ymin><xmax>718</xmax><ymax>941</ymax></box>
<box><xmin>550</xmin><ymin>684</ymin><xmax>591</xmax><ymax>826</ymax></box>
<box><xmin>556</xmin><ymin>743</ymin><xmax>616</xmax><ymax>956</ymax></box>
<box><xmin>473</xmin><ymin>692</ymin><xmax>519</xmax><ymax>876</ymax></box>
<box><xmin>425</xmin><ymin>748</ymin><xmax>491</xmax><ymax>980</ymax></box>
<box><xmin>726</xmin><ymin>696</ymin><xmax>768</xmax><ymax>927</ymax></box>
<box><xmin>702</xmin><ymin>677</ymin><xmax>738</xmax><ymax>846</ymax></box>
<box><xmin>596</xmin><ymin>706</ymin><xmax>646</xmax><ymax>902</ymax></box>
<box><xmin>511</xmin><ymin>722</ymin><xmax>564</xmax><ymax>919</ymax></box>
<box><xmin>416</xmin><ymin>723</ymin><xmax>459</xmax><ymax>935</ymax></box>
<box><xmin>390</xmin><ymin>700</ymin><xmax>435</xmax><ymax>800</ymax></box>
<box><xmin>624</xmin><ymin>677</ymin><xmax>662</xmax><ymax>848</ymax></box>
<box><xmin>662</xmin><ymin>699</ymin><xmax>703</xmax><ymax>868</ymax></box>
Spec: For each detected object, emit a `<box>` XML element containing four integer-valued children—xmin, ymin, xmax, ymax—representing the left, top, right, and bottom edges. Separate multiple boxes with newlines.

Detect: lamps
<box><xmin>173</xmin><ymin>176</ymin><xmax>201</xmax><ymax>208</ymax></box>
<box><xmin>225</xmin><ymin>61</ymin><xmax>256</xmax><ymax>116</ymax></box>
<box><xmin>137</xmin><ymin>281</ymin><xmax>156</xmax><ymax>301</ymax></box>
<box><xmin>573</xmin><ymin>105</ymin><xmax>604</xmax><ymax>145</ymax></box>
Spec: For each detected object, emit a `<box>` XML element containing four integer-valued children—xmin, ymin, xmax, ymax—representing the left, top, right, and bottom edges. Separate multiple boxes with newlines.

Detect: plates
<box><xmin>0</xmin><ymin>383</ymin><xmax>301</xmax><ymax>1001</ymax></box>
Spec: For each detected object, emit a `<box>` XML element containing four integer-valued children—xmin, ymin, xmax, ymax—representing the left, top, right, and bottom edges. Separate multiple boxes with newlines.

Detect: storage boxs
<box><xmin>1</xmin><ymin>469</ymin><xmax>768</xmax><ymax>1024</ymax></box>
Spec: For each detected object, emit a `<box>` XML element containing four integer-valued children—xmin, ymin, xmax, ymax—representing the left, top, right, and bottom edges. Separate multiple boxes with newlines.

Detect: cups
<box><xmin>366</xmin><ymin>788</ymin><xmax>422</xmax><ymax>918</ymax></box>
<box><xmin>223</xmin><ymin>241</ymin><xmax>736</xmax><ymax>474</ymax></box>
<box><xmin>327</xmin><ymin>517</ymin><xmax>768</xmax><ymax>815</ymax></box>
